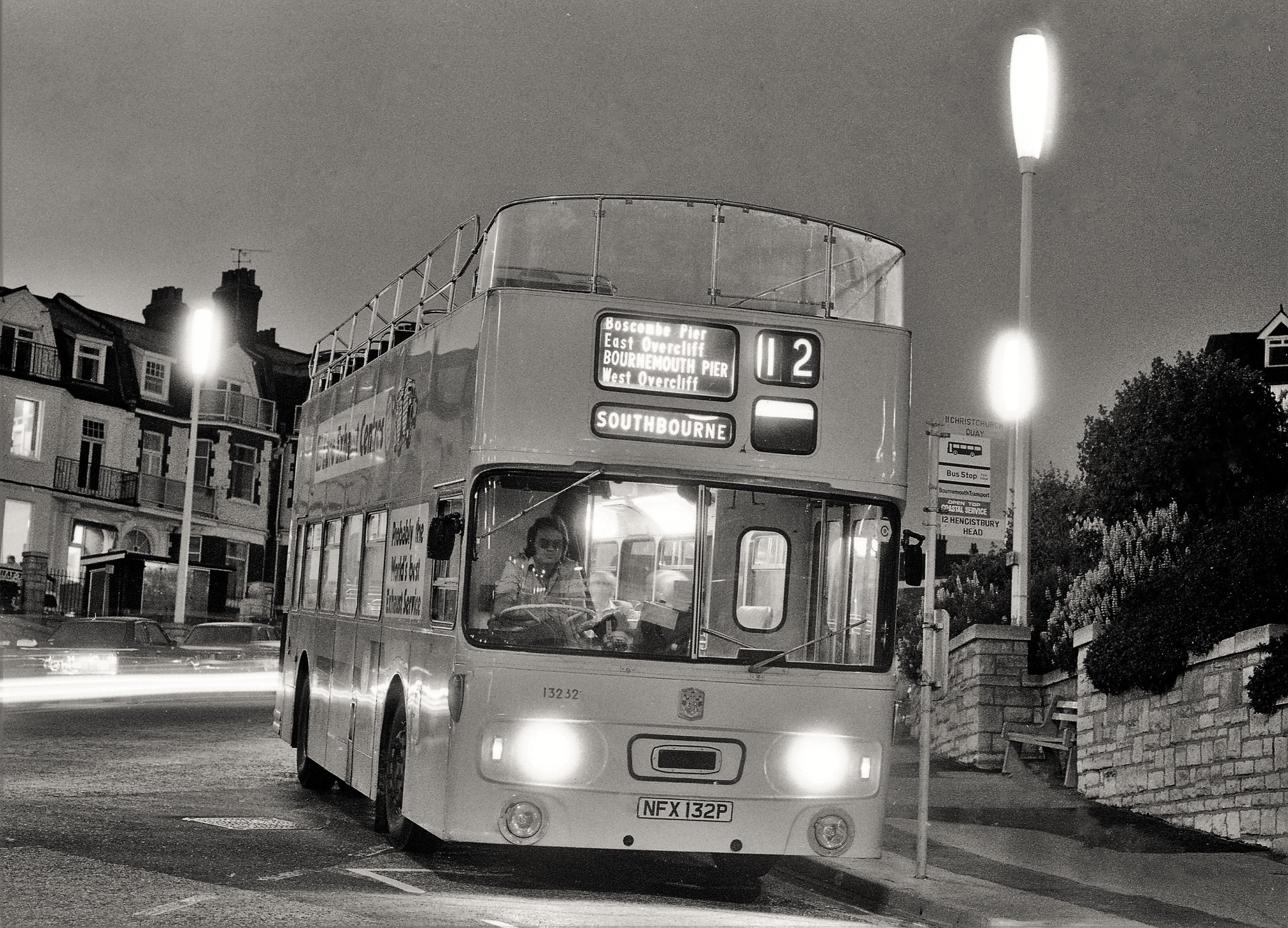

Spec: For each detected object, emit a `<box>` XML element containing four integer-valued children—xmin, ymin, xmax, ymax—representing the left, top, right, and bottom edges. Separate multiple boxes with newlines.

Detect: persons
<box><xmin>493</xmin><ymin>516</ymin><xmax>587</xmax><ymax>631</ymax></box>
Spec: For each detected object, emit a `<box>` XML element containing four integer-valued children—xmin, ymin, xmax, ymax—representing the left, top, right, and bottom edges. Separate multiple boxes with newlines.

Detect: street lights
<box><xmin>171</xmin><ymin>308</ymin><xmax>223</xmax><ymax>624</ymax></box>
<box><xmin>986</xmin><ymin>328</ymin><xmax>1036</xmax><ymax>529</ymax></box>
<box><xmin>1006</xmin><ymin>27</ymin><xmax>1054</xmax><ymax>626</ymax></box>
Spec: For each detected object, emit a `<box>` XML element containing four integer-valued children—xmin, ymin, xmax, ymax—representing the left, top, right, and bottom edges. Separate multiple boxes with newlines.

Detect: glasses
<box><xmin>532</xmin><ymin>538</ymin><xmax>565</xmax><ymax>548</ymax></box>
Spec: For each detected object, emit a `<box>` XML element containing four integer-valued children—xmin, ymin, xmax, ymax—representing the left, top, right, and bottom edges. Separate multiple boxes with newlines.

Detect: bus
<box><xmin>272</xmin><ymin>190</ymin><xmax>927</xmax><ymax>878</ymax></box>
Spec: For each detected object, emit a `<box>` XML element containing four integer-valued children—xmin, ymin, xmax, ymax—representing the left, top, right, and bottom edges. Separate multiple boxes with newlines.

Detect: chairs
<box><xmin>735</xmin><ymin>600</ymin><xmax>775</xmax><ymax>632</ymax></box>
<box><xmin>342</xmin><ymin>586</ymin><xmax>357</xmax><ymax>616</ymax></box>
<box><xmin>367</xmin><ymin>597</ymin><xmax>380</xmax><ymax>616</ymax></box>
<box><xmin>586</xmin><ymin>568</ymin><xmax>619</xmax><ymax>614</ymax></box>
<box><xmin>647</xmin><ymin>569</ymin><xmax>687</xmax><ymax>605</ymax></box>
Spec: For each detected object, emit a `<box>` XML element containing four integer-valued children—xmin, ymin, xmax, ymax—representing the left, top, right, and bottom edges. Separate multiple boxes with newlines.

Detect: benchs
<box><xmin>998</xmin><ymin>694</ymin><xmax>1081</xmax><ymax>791</ymax></box>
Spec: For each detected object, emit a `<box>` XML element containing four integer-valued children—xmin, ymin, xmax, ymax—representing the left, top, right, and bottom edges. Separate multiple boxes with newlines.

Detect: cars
<box><xmin>0</xmin><ymin>614</ymin><xmax>279</xmax><ymax>680</ymax></box>
